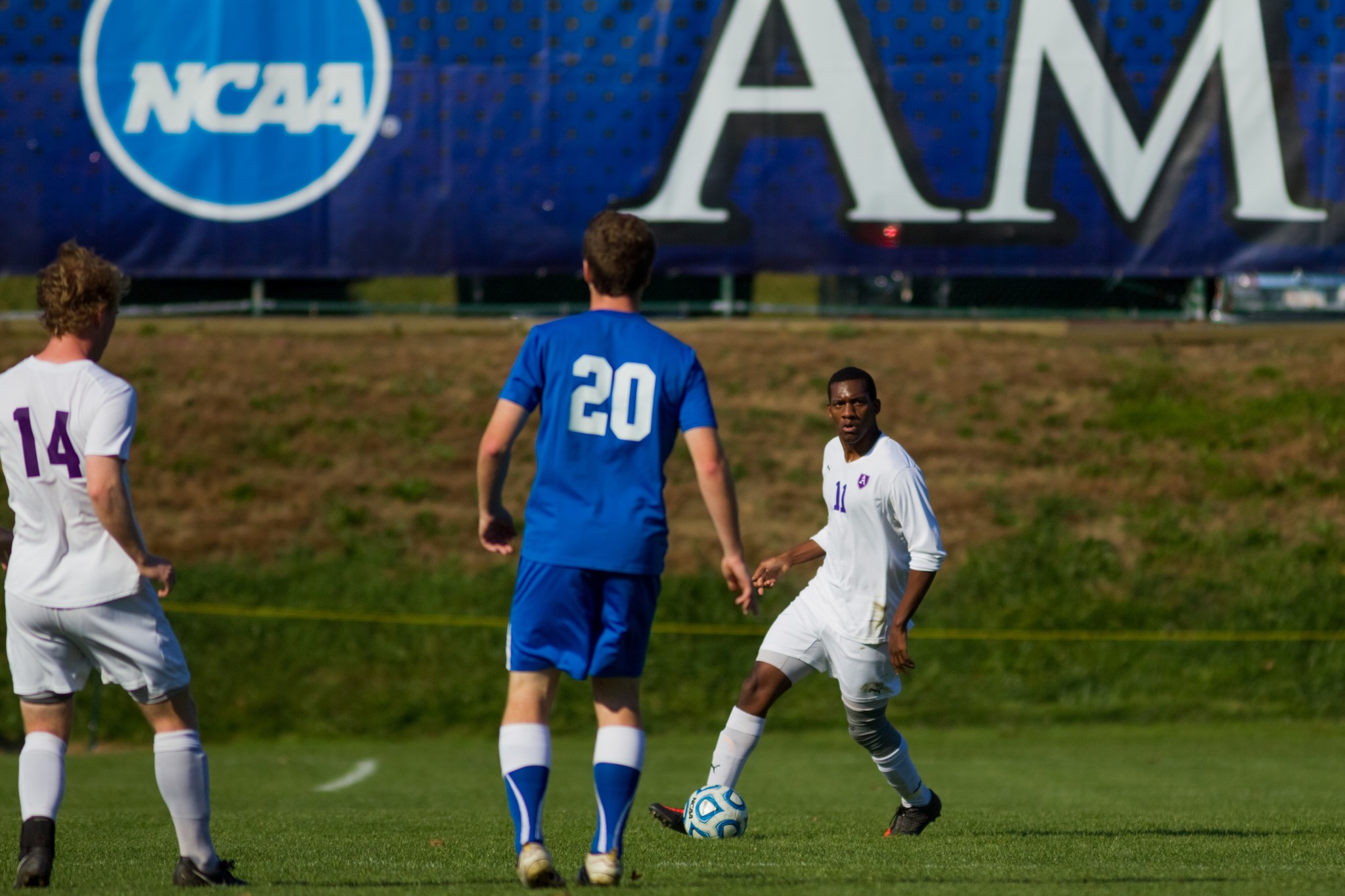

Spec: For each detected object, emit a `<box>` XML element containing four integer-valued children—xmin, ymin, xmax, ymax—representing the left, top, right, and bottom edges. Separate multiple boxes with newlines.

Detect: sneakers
<box><xmin>883</xmin><ymin>789</ymin><xmax>941</xmax><ymax>836</ymax></box>
<box><xmin>514</xmin><ymin>841</ymin><xmax>565</xmax><ymax>886</ymax></box>
<box><xmin>580</xmin><ymin>850</ymin><xmax>622</xmax><ymax>886</ymax></box>
<box><xmin>175</xmin><ymin>855</ymin><xmax>246</xmax><ymax>885</ymax></box>
<box><xmin>11</xmin><ymin>848</ymin><xmax>53</xmax><ymax>888</ymax></box>
<box><xmin>648</xmin><ymin>802</ymin><xmax>687</xmax><ymax>834</ymax></box>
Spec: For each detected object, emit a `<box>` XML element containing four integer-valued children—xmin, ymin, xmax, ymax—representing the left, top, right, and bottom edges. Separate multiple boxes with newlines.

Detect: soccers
<box><xmin>682</xmin><ymin>784</ymin><xmax>748</xmax><ymax>839</ymax></box>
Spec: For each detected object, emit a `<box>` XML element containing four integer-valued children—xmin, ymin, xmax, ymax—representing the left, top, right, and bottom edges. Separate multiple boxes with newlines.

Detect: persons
<box><xmin>648</xmin><ymin>368</ymin><xmax>946</xmax><ymax>838</ymax></box>
<box><xmin>474</xmin><ymin>213</ymin><xmax>758</xmax><ymax>886</ymax></box>
<box><xmin>1</xmin><ymin>242</ymin><xmax>250</xmax><ymax>887</ymax></box>
